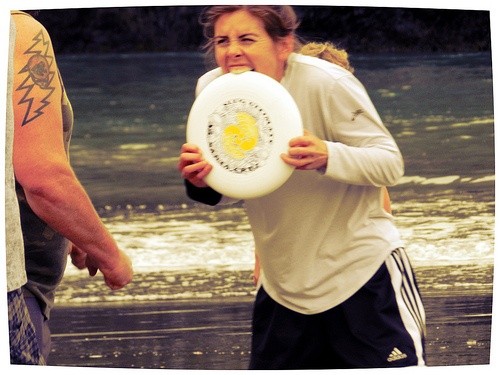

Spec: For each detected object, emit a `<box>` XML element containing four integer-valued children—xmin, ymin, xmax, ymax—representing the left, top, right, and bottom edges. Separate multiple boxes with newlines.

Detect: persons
<box><xmin>177</xmin><ymin>6</ymin><xmax>428</xmax><ymax>368</ymax></box>
<box><xmin>7</xmin><ymin>9</ymin><xmax>135</xmax><ymax>366</ymax></box>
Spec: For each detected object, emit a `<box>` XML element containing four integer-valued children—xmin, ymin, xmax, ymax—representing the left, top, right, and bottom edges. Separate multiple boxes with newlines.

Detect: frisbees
<box><xmin>185</xmin><ymin>69</ymin><xmax>303</xmax><ymax>201</ymax></box>
